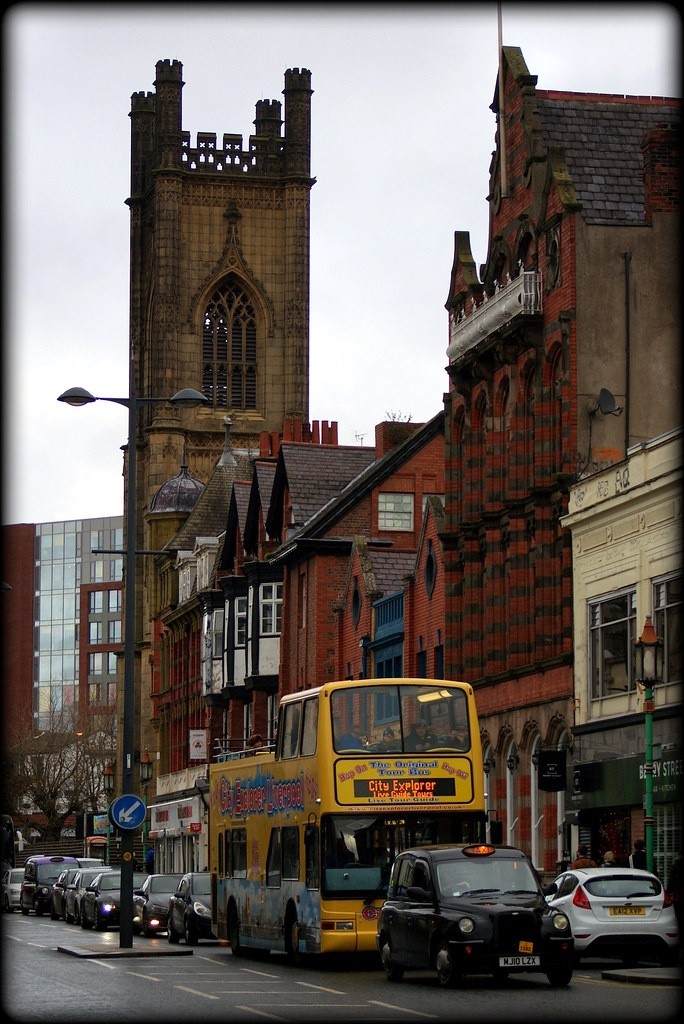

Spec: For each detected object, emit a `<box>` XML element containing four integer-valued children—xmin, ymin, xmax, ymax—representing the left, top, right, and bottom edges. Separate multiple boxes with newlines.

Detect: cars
<box><xmin>19</xmin><ymin>855</ymin><xmax>81</xmax><ymax>917</ymax></box>
<box><xmin>49</xmin><ymin>866</ymin><xmax>120</xmax><ymax>925</ymax></box>
<box><xmin>168</xmin><ymin>869</ymin><xmax>212</xmax><ymax>945</ymax></box>
<box><xmin>1</xmin><ymin>868</ymin><xmax>27</xmax><ymax>913</ymax></box>
<box><xmin>544</xmin><ymin>867</ymin><xmax>677</xmax><ymax>968</ymax></box>
<box><xmin>133</xmin><ymin>874</ymin><xmax>182</xmax><ymax>938</ymax></box>
<box><xmin>75</xmin><ymin>857</ymin><xmax>105</xmax><ymax>869</ymax></box>
<box><xmin>80</xmin><ymin>872</ymin><xmax>148</xmax><ymax>931</ymax></box>
<box><xmin>377</xmin><ymin>845</ymin><xmax>576</xmax><ymax>988</ymax></box>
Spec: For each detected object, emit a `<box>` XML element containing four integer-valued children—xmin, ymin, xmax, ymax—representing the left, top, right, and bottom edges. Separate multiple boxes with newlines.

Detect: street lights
<box><xmin>56</xmin><ymin>386</ymin><xmax>215</xmax><ymax>946</ymax></box>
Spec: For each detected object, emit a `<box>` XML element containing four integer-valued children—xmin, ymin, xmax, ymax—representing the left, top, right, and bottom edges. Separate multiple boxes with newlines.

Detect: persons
<box><xmin>243</xmin><ymin>719</ymin><xmax>437</xmax><ymax>758</ymax></box>
<box><xmin>131</xmin><ymin>847</ymin><xmax>153</xmax><ymax>875</ymax></box>
<box><xmin>573</xmin><ymin>838</ymin><xmax>657</xmax><ymax>875</ymax></box>
<box><xmin>665</xmin><ymin>848</ymin><xmax>680</xmax><ymax>953</ymax></box>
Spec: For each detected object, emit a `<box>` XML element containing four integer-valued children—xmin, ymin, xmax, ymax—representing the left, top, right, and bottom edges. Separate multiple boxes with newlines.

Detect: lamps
<box><xmin>483</xmin><ymin>758</ymin><xmax>496</xmax><ymax>779</ymax></box>
<box><xmin>532</xmin><ymin>749</ymin><xmax>542</xmax><ymax>771</ymax></box>
<box><xmin>506</xmin><ymin>755</ymin><xmax>520</xmax><ymax>775</ymax></box>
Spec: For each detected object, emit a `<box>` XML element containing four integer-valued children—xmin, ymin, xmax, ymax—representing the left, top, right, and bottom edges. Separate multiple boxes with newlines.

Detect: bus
<box><xmin>0</xmin><ymin>815</ymin><xmax>25</xmax><ymax>872</ymax></box>
<box><xmin>209</xmin><ymin>680</ymin><xmax>504</xmax><ymax>969</ymax></box>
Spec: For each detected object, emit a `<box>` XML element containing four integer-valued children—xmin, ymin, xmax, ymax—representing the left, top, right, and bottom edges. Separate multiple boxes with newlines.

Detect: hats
<box><xmin>414</xmin><ymin>719</ymin><xmax>427</xmax><ymax>729</ymax></box>
<box><xmin>384</xmin><ymin>727</ymin><xmax>395</xmax><ymax>739</ymax></box>
